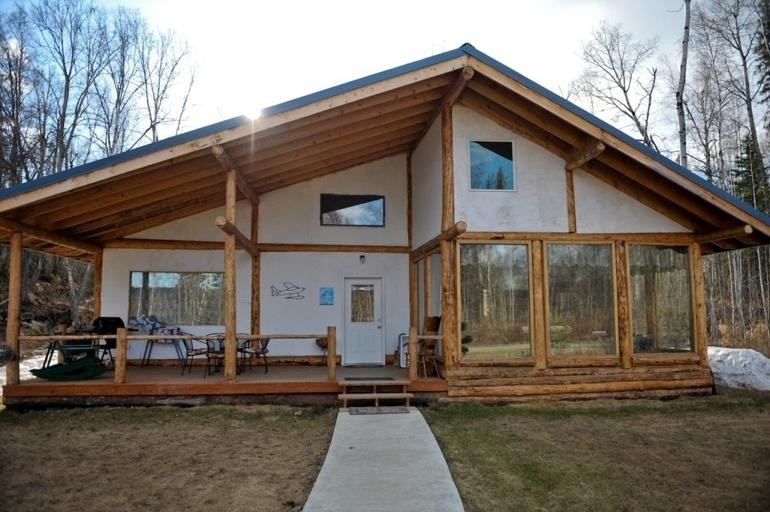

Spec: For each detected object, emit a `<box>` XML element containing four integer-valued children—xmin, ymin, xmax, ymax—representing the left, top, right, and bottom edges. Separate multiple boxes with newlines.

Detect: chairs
<box><xmin>403</xmin><ymin>314</ymin><xmax>442</xmax><ymax>380</ymax></box>
<box><xmin>179</xmin><ymin>331</ymin><xmax>270</xmax><ymax>379</ymax></box>
